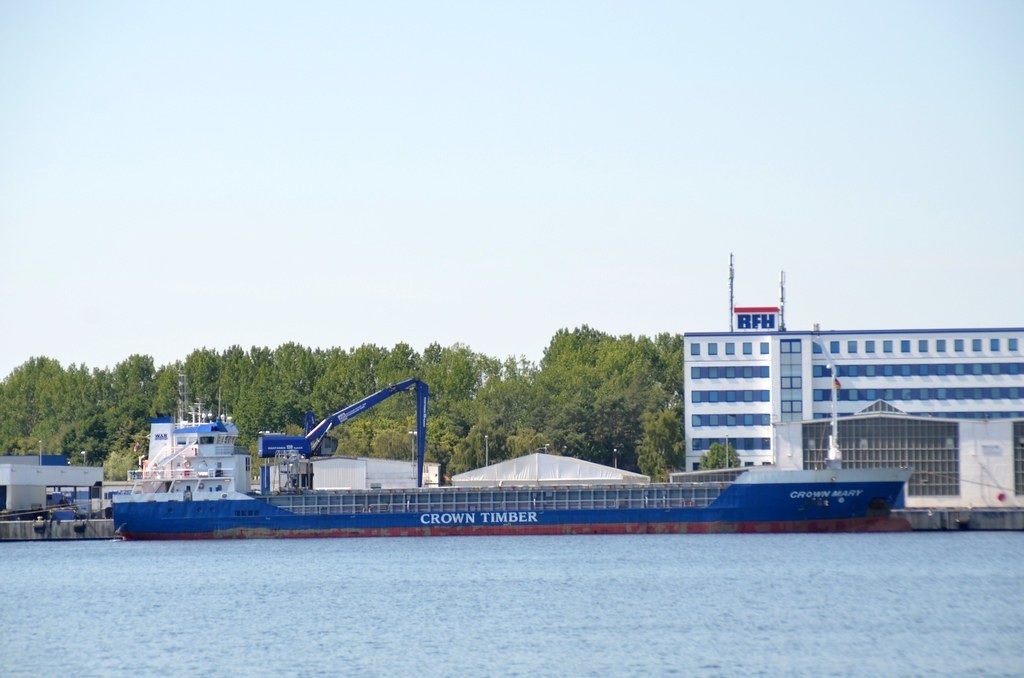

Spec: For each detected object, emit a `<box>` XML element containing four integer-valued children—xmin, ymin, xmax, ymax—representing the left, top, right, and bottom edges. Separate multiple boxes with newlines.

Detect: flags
<box><xmin>833</xmin><ymin>378</ymin><xmax>841</xmax><ymax>388</ymax></box>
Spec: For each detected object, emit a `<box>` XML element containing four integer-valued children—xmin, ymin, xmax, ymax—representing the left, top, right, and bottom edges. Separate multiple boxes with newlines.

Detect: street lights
<box><xmin>408</xmin><ymin>431</ymin><xmax>418</xmax><ymax>466</ymax></box>
<box><xmin>81</xmin><ymin>450</ymin><xmax>89</xmax><ymax>466</ymax></box>
<box><xmin>545</xmin><ymin>443</ymin><xmax>550</xmax><ymax>453</ymax></box>
<box><xmin>614</xmin><ymin>448</ymin><xmax>618</xmax><ymax>468</ymax></box>
<box><xmin>38</xmin><ymin>439</ymin><xmax>43</xmax><ymax>466</ymax></box>
<box><xmin>485</xmin><ymin>435</ymin><xmax>489</xmax><ymax>467</ymax></box>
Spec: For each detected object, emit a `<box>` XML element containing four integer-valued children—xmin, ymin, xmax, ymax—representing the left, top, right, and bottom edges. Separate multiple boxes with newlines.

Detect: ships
<box><xmin>109</xmin><ymin>359</ymin><xmax>915</xmax><ymax>546</ymax></box>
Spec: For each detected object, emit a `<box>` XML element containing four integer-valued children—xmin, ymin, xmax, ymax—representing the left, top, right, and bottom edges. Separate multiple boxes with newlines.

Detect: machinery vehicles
<box><xmin>258</xmin><ymin>377</ymin><xmax>429</xmax><ymax>486</ymax></box>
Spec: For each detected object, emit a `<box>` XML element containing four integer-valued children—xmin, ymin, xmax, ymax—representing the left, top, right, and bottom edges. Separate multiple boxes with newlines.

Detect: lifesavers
<box><xmin>184</xmin><ymin>461</ymin><xmax>191</xmax><ymax>468</ymax></box>
